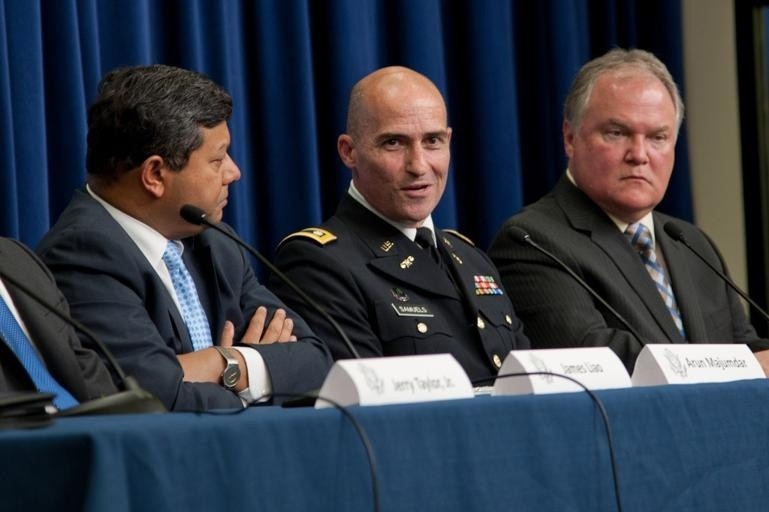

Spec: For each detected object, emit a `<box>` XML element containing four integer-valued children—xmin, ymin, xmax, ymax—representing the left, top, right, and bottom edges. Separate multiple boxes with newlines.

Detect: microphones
<box><xmin>505</xmin><ymin>225</ymin><xmax>646</xmax><ymax>347</ymax></box>
<box><xmin>0</xmin><ymin>271</ymin><xmax>168</xmax><ymax>416</ymax></box>
<box><xmin>179</xmin><ymin>204</ymin><xmax>363</xmax><ymax>408</ymax></box>
<box><xmin>662</xmin><ymin>222</ymin><xmax>769</xmax><ymax>322</ymax></box>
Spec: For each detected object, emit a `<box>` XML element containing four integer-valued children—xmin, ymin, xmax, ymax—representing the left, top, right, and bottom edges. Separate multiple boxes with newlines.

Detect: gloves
<box><xmin>0</xmin><ymin>296</ymin><xmax>80</xmax><ymax>412</ymax></box>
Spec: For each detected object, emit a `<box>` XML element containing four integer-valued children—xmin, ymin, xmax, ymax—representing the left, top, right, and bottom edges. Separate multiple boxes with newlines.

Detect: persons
<box><xmin>263</xmin><ymin>64</ymin><xmax>532</xmax><ymax>388</ymax></box>
<box><xmin>486</xmin><ymin>47</ymin><xmax>769</xmax><ymax>381</ymax></box>
<box><xmin>0</xmin><ymin>235</ymin><xmax>118</xmax><ymax>414</ymax></box>
<box><xmin>34</xmin><ymin>63</ymin><xmax>336</xmax><ymax>415</ymax></box>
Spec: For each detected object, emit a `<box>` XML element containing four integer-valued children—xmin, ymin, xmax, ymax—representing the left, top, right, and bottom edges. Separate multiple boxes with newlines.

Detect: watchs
<box><xmin>211</xmin><ymin>345</ymin><xmax>241</xmax><ymax>393</ymax></box>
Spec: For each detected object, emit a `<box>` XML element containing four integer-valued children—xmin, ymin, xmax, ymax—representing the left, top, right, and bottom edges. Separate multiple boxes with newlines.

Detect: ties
<box><xmin>162</xmin><ymin>239</ymin><xmax>215</xmax><ymax>353</ymax></box>
<box><xmin>626</xmin><ymin>222</ymin><xmax>687</xmax><ymax>344</ymax></box>
<box><xmin>413</xmin><ymin>227</ymin><xmax>465</xmax><ymax>302</ymax></box>
